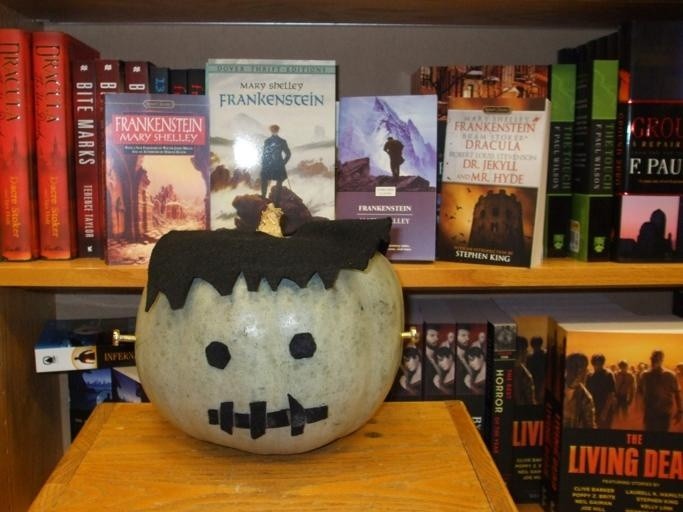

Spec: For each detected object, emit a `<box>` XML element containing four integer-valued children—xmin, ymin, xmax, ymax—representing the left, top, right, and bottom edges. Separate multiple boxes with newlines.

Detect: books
<box><xmin>382</xmin><ymin>293</ymin><xmax>683</xmax><ymax>511</ymax></box>
<box><xmin>33</xmin><ymin>315</ymin><xmax>150</xmax><ymax>443</ymax></box>
<box><xmin>1</xmin><ymin>28</ymin><xmax>682</xmax><ymax>269</ymax></box>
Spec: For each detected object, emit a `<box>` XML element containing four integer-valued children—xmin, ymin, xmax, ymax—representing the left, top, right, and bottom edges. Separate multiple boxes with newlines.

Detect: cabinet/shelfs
<box><xmin>0</xmin><ymin>0</ymin><xmax>683</xmax><ymax>512</ymax></box>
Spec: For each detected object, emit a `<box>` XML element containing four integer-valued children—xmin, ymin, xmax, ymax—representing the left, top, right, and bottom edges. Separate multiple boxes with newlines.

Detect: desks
<box><xmin>27</xmin><ymin>400</ymin><xmax>521</xmax><ymax>511</ymax></box>
